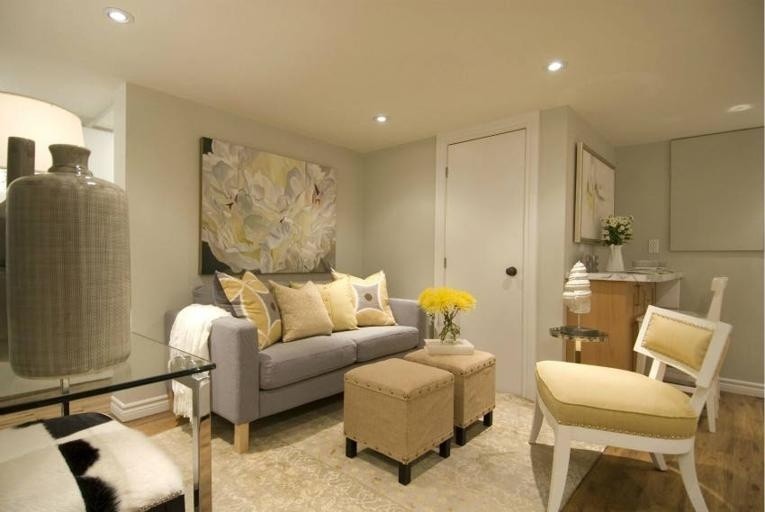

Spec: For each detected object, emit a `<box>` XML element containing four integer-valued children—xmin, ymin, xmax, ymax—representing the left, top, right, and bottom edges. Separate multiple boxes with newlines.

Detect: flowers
<box><xmin>416</xmin><ymin>286</ymin><xmax>476</xmax><ymax>344</ymax></box>
<box><xmin>597</xmin><ymin>213</ymin><xmax>634</xmax><ymax>246</ymax></box>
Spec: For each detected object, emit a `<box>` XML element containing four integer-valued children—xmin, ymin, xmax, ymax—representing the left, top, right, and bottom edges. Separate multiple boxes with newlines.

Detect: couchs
<box><xmin>162</xmin><ymin>279</ymin><xmax>427</xmax><ymax>454</ymax></box>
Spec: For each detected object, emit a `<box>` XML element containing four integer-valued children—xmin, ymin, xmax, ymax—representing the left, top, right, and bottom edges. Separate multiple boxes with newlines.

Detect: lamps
<box><xmin>548</xmin><ymin>259</ymin><xmax>611</xmax><ymax>364</ymax></box>
<box><xmin>0</xmin><ymin>90</ymin><xmax>85</xmax><ymax>362</ymax></box>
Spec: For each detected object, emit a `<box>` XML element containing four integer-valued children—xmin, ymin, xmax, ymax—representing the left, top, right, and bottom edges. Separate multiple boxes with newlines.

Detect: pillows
<box><xmin>268</xmin><ymin>278</ymin><xmax>333</xmax><ymax>343</ymax></box>
<box><xmin>211</xmin><ymin>268</ymin><xmax>283</xmax><ymax>350</ymax></box>
<box><xmin>329</xmin><ymin>267</ymin><xmax>399</xmax><ymax>327</ymax></box>
<box><xmin>290</xmin><ymin>273</ymin><xmax>355</xmax><ymax>331</ymax></box>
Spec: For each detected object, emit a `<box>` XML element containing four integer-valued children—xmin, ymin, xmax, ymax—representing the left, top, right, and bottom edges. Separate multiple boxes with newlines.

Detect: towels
<box><xmin>167</xmin><ymin>301</ymin><xmax>233</xmax><ymax>420</ymax></box>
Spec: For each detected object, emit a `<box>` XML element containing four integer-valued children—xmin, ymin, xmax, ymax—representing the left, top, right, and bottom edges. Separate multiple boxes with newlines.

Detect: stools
<box><xmin>405</xmin><ymin>347</ymin><xmax>496</xmax><ymax>446</ymax></box>
<box><xmin>343</xmin><ymin>358</ymin><xmax>454</xmax><ymax>485</ymax></box>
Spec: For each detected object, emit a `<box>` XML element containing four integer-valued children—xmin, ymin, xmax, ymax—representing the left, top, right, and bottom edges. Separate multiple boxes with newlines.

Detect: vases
<box><xmin>606</xmin><ymin>244</ymin><xmax>624</xmax><ymax>272</ymax></box>
<box><xmin>6</xmin><ymin>143</ymin><xmax>131</xmax><ymax>378</ymax></box>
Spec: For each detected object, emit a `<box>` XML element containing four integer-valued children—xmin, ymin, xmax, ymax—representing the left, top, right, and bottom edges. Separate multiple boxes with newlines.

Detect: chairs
<box><xmin>0</xmin><ymin>413</ymin><xmax>186</xmax><ymax>512</ymax></box>
<box><xmin>636</xmin><ymin>275</ymin><xmax>728</xmax><ymax>432</ymax></box>
<box><xmin>528</xmin><ymin>304</ymin><xmax>734</xmax><ymax>512</ymax></box>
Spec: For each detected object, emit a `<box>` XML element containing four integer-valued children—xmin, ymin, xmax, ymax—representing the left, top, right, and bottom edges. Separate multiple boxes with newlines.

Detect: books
<box><xmin>625</xmin><ymin>257</ymin><xmax>672</xmax><ymax>274</ymax></box>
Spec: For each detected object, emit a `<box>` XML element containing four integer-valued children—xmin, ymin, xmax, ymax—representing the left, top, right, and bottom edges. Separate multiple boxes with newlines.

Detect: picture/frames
<box><xmin>574</xmin><ymin>142</ymin><xmax>617</xmax><ymax>245</ymax></box>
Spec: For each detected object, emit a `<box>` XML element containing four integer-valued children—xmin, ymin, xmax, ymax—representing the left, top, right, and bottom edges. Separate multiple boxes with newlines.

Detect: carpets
<box><xmin>150</xmin><ymin>385</ymin><xmax>608</xmax><ymax>512</ymax></box>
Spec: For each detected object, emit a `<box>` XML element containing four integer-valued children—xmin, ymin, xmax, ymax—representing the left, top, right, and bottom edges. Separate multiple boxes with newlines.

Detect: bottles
<box><xmin>0</xmin><ymin>132</ymin><xmax>133</xmax><ymax>380</ymax></box>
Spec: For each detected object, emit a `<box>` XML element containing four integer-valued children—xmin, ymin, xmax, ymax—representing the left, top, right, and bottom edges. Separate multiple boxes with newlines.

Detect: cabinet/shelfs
<box><xmin>563</xmin><ymin>279</ymin><xmax>656</xmax><ymax>371</ymax></box>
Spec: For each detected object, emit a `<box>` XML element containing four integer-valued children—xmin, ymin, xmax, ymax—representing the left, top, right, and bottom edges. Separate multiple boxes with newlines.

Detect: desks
<box><xmin>0</xmin><ymin>331</ymin><xmax>216</xmax><ymax>512</ymax></box>
